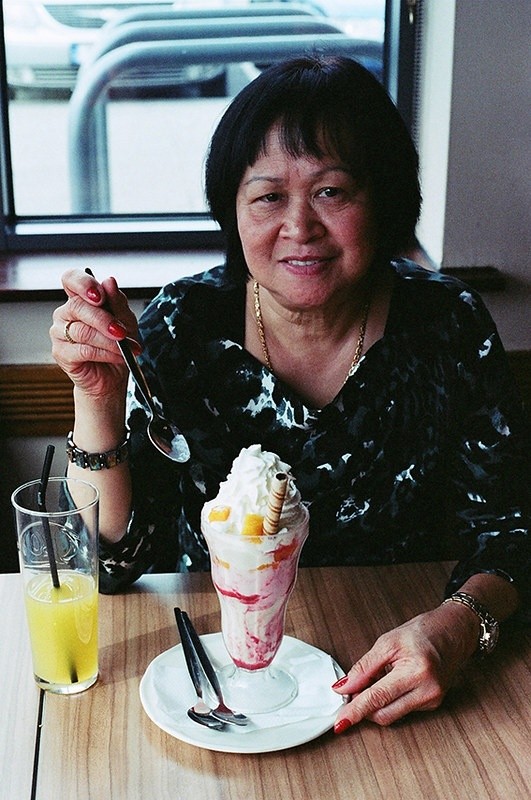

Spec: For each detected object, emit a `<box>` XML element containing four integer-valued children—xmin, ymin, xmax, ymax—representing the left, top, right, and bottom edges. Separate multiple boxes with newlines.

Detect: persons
<box><xmin>49</xmin><ymin>55</ymin><xmax>531</xmax><ymax>736</ymax></box>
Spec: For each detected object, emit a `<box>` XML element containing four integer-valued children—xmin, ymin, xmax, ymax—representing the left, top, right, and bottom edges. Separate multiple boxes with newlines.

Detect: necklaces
<box><xmin>254</xmin><ymin>281</ymin><xmax>368</xmax><ymax>374</ymax></box>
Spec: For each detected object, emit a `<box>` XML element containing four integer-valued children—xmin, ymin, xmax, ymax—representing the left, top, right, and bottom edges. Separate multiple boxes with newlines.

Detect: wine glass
<box><xmin>201</xmin><ymin>502</ymin><xmax>310</xmax><ymax>714</ymax></box>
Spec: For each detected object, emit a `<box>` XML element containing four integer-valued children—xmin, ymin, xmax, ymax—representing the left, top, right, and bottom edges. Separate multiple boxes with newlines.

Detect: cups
<box><xmin>11</xmin><ymin>477</ymin><xmax>100</xmax><ymax>695</ymax></box>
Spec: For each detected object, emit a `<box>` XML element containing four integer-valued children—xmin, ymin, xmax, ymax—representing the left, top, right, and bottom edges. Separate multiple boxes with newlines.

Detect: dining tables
<box><xmin>0</xmin><ymin>561</ymin><xmax>531</xmax><ymax>800</ymax></box>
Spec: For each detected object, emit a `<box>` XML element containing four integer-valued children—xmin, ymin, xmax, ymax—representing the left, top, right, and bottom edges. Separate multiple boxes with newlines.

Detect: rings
<box><xmin>65</xmin><ymin>320</ymin><xmax>81</xmax><ymax>344</ymax></box>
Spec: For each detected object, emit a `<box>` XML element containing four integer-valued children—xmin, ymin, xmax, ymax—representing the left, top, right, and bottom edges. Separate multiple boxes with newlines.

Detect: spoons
<box><xmin>173</xmin><ymin>607</ymin><xmax>228</xmax><ymax>731</ymax></box>
<box><xmin>181</xmin><ymin>610</ymin><xmax>250</xmax><ymax>727</ymax></box>
<box><xmin>84</xmin><ymin>267</ymin><xmax>191</xmax><ymax>463</ymax></box>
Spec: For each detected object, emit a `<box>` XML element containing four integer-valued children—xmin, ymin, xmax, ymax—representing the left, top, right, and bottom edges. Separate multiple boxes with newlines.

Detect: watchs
<box><xmin>440</xmin><ymin>592</ymin><xmax>499</xmax><ymax>662</ymax></box>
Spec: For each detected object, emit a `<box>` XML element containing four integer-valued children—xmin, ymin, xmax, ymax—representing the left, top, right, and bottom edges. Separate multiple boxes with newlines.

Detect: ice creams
<box><xmin>201</xmin><ymin>444</ymin><xmax>308</xmax><ymax>670</ymax></box>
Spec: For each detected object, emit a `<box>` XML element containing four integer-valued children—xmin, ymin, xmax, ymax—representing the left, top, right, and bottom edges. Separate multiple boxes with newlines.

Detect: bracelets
<box><xmin>66</xmin><ymin>425</ymin><xmax>131</xmax><ymax>472</ymax></box>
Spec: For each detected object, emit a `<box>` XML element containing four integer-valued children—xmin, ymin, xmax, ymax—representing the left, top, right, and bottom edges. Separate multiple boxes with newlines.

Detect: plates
<box><xmin>140</xmin><ymin>631</ymin><xmax>353</xmax><ymax>754</ymax></box>
